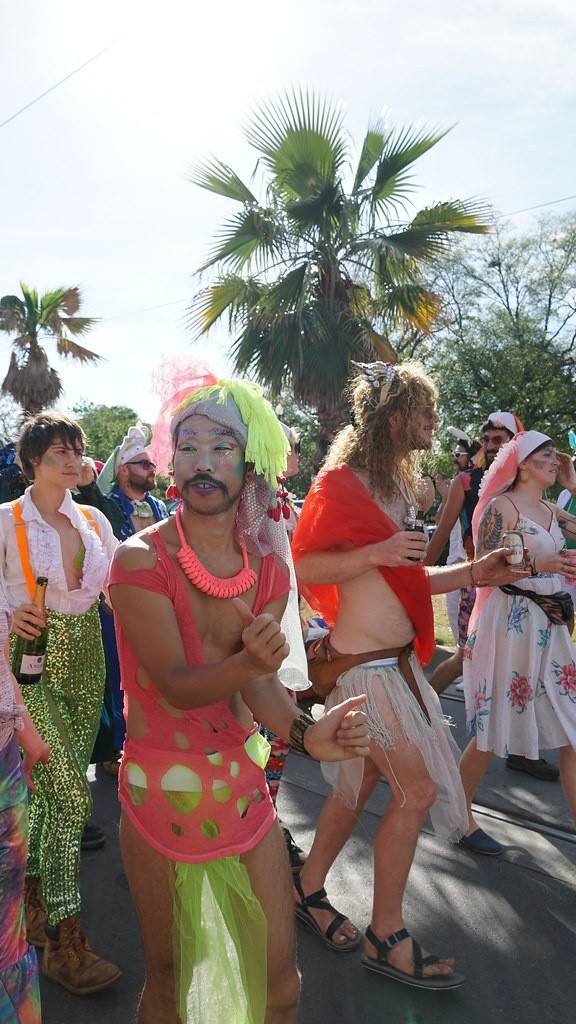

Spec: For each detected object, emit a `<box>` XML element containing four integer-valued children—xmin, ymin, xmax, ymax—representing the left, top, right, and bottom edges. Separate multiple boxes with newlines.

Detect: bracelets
<box><xmin>290</xmin><ymin>714</ymin><xmax>316</xmax><ymax>759</ymax></box>
<box><xmin>469</xmin><ymin>561</ymin><xmax>489</xmax><ymax>587</ymax></box>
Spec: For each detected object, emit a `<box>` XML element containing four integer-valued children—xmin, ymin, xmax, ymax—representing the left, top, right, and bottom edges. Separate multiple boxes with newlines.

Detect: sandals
<box><xmin>360</xmin><ymin>924</ymin><xmax>465</xmax><ymax>991</ymax></box>
<box><xmin>291</xmin><ymin>875</ymin><xmax>363</xmax><ymax>951</ymax></box>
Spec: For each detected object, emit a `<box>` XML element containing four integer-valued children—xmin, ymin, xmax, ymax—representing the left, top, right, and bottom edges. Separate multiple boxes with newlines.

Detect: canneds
<box><xmin>502</xmin><ymin>530</ymin><xmax>526</xmax><ymax>569</ymax></box>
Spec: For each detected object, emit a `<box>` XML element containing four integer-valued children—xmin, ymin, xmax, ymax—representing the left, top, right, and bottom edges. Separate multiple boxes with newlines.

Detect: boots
<box><xmin>24</xmin><ymin>877</ymin><xmax>47</xmax><ymax>945</ymax></box>
<box><xmin>41</xmin><ymin>914</ymin><xmax>122</xmax><ymax>994</ymax></box>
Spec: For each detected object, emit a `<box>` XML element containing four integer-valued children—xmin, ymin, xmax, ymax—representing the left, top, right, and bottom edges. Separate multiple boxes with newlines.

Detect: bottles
<box><xmin>11</xmin><ymin>576</ymin><xmax>49</xmax><ymax>685</ymax></box>
<box><xmin>406</xmin><ymin>510</ymin><xmax>426</xmax><ymax>563</ymax></box>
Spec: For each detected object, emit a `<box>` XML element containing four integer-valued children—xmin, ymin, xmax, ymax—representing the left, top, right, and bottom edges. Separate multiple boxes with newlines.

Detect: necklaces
<box><xmin>394</xmin><ymin>477</ymin><xmax>416</xmax><ymax>528</ymax></box>
<box><xmin>126</xmin><ymin>493</ymin><xmax>154</xmax><ymax>518</ymax></box>
<box><xmin>176</xmin><ymin>507</ymin><xmax>257</xmax><ymax>598</ymax></box>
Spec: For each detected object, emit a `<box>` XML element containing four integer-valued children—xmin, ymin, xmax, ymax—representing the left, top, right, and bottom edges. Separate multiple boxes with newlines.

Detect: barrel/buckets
<box><xmin>427</xmin><ymin>525</ymin><xmax>450</xmax><ymax>567</ymax></box>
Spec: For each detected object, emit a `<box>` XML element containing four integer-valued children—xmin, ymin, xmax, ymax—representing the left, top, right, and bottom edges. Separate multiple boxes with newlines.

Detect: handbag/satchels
<box><xmin>306</xmin><ymin>633</ymin><xmax>431</xmax><ymax>726</ymax></box>
<box><xmin>500</xmin><ymin>585</ymin><xmax>574</xmax><ymax>625</ymax></box>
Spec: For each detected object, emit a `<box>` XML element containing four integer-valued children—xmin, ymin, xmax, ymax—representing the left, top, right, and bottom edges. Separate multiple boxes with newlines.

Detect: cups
<box><xmin>563</xmin><ymin>549</ymin><xmax>576</xmax><ymax>586</ymax></box>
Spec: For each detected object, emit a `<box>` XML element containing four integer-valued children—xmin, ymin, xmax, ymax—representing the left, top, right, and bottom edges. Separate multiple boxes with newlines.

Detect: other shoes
<box><xmin>81</xmin><ymin>825</ymin><xmax>106</xmax><ymax>848</ymax></box>
<box><xmin>455</xmin><ymin>828</ymin><xmax>505</xmax><ymax>854</ymax></box>
<box><xmin>277</xmin><ymin>818</ymin><xmax>309</xmax><ymax>875</ymax></box>
<box><xmin>506</xmin><ymin>752</ymin><xmax>559</xmax><ymax>778</ymax></box>
<box><xmin>452</xmin><ymin>675</ymin><xmax>463</xmax><ymax>690</ymax></box>
<box><xmin>103</xmin><ymin>753</ymin><xmax>123</xmax><ymax>775</ymax></box>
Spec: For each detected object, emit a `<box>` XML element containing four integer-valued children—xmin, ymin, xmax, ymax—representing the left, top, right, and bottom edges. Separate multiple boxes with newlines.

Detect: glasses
<box><xmin>126</xmin><ymin>460</ymin><xmax>157</xmax><ymax>470</ymax></box>
<box><xmin>288</xmin><ymin>442</ymin><xmax>301</xmax><ymax>454</ymax></box>
<box><xmin>479</xmin><ymin>435</ymin><xmax>505</xmax><ymax>445</ymax></box>
<box><xmin>454</xmin><ymin>453</ymin><xmax>470</xmax><ymax>459</ymax></box>
<box><xmin>412</xmin><ymin>402</ymin><xmax>435</xmax><ymax>415</ymax></box>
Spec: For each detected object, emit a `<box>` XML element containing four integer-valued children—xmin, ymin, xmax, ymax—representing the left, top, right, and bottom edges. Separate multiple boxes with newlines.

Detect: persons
<box><xmin>416</xmin><ymin>410</ymin><xmax>574</xmax><ymax>780</ymax></box>
<box><xmin>292</xmin><ymin>365</ymin><xmax>529</xmax><ymax>987</ymax></box>
<box><xmin>108</xmin><ymin>364</ymin><xmax>369</xmax><ymax>1024</ymax></box>
<box><xmin>459</xmin><ymin>430</ymin><xmax>575</xmax><ymax>853</ymax></box>
<box><xmin>0</xmin><ymin>592</ymin><xmax>49</xmax><ymax>1024</ymax></box>
<box><xmin>0</xmin><ymin>422</ymin><xmax>311</xmax><ymax>871</ymax></box>
<box><xmin>0</xmin><ymin>411</ymin><xmax>122</xmax><ymax>993</ymax></box>
